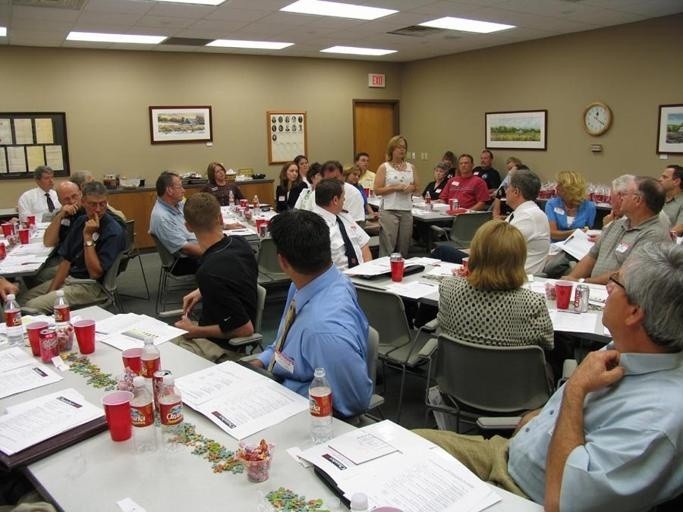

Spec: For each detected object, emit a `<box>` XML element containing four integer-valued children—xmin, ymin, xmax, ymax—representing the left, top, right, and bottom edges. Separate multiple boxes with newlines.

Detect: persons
<box><xmin>342</xmin><ymin>164</ymin><xmax>374</xmax><ymax>214</ymax></box>
<box><xmin>200</xmin><ymin>160</ymin><xmax>244</xmax><ymax>207</ymax></box>
<box><xmin>149</xmin><ymin>173</ymin><xmax>202</xmax><ymax>275</ymax></box>
<box><xmin>292</xmin><ymin>162</ymin><xmax>322</xmax><ymax>212</ymax></box>
<box><xmin>0</xmin><ymin>275</ymin><xmax>19</xmax><ymax>302</ymax></box>
<box><xmin>17</xmin><ymin>180</ymin><xmax>124</xmax><ymax>315</ymax></box>
<box><xmin>18</xmin><ymin>166</ymin><xmax>62</xmax><ymax>222</ymax></box>
<box><xmin>71</xmin><ymin>171</ymin><xmax>126</xmax><ymax>221</ymax></box>
<box><xmin>274</xmin><ymin>161</ymin><xmax>308</xmax><ymax>211</ymax></box>
<box><xmin>411</xmin><ymin>241</ymin><xmax>683</xmax><ymax>512</ymax></box>
<box><xmin>247</xmin><ymin>210</ymin><xmax>374</xmax><ymax>421</ymax></box>
<box><xmin>437</xmin><ymin>154</ymin><xmax>490</xmax><ymax>211</ymax></box>
<box><xmin>472</xmin><ymin>150</ymin><xmax>501</xmax><ymax>193</ymax></box>
<box><xmin>544</xmin><ymin>171</ymin><xmax>593</xmax><ymax>239</ymax></box>
<box><xmin>421</xmin><ymin>162</ymin><xmax>449</xmax><ymax>199</ymax></box>
<box><xmin>31</xmin><ymin>181</ymin><xmax>124</xmax><ymax>284</ymax></box>
<box><xmin>320</xmin><ymin>159</ymin><xmax>366</xmax><ymax>223</ymax></box>
<box><xmin>659</xmin><ymin>164</ymin><xmax>683</xmax><ymax>236</ymax></box>
<box><xmin>601</xmin><ymin>175</ymin><xmax>633</xmax><ymax>224</ymax></box>
<box><xmin>312</xmin><ymin>178</ymin><xmax>373</xmax><ymax>275</ymax></box>
<box><xmin>430</xmin><ymin>170</ymin><xmax>550</xmax><ymax>274</ymax></box>
<box><xmin>504</xmin><ymin>156</ymin><xmax>521</xmax><ymax>170</ymax></box>
<box><xmin>490</xmin><ymin>165</ymin><xmax>529</xmax><ymax>222</ymax></box>
<box><xmin>373</xmin><ymin>135</ymin><xmax>420</xmax><ymax>257</ymax></box>
<box><xmin>559</xmin><ymin>176</ymin><xmax>672</xmax><ymax>285</ymax></box>
<box><xmin>354</xmin><ymin>152</ymin><xmax>376</xmax><ymax>189</ymax></box>
<box><xmin>441</xmin><ymin>151</ymin><xmax>459</xmax><ymax>180</ymax></box>
<box><xmin>175</xmin><ymin>192</ymin><xmax>258</xmax><ymax>363</ymax></box>
<box><xmin>294</xmin><ymin>155</ymin><xmax>311</xmax><ymax>188</ymax></box>
<box><xmin>434</xmin><ymin>220</ymin><xmax>556</xmax><ymax>419</ymax></box>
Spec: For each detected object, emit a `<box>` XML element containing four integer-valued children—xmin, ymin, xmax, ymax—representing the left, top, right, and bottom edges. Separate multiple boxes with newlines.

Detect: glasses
<box><xmin>608</xmin><ymin>273</ymin><xmax>626</xmax><ymax>289</ymax></box>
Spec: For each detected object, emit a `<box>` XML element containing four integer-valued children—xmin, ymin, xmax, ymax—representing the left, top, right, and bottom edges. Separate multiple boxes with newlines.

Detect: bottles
<box><xmin>425</xmin><ymin>191</ymin><xmax>431</xmax><ymax>204</ymax></box>
<box><xmin>390</xmin><ymin>253</ymin><xmax>402</xmax><ymax>261</ymax></box>
<box><xmin>308</xmin><ymin>368</ymin><xmax>333</xmax><ymax>445</ymax></box>
<box><xmin>229</xmin><ymin>191</ymin><xmax>234</xmax><ymax>206</ymax></box>
<box><xmin>152</xmin><ymin>369</ymin><xmax>173</xmax><ymax>412</ymax></box>
<box><xmin>129</xmin><ymin>376</ymin><xmax>157</xmax><ymax>454</ymax></box>
<box><xmin>252</xmin><ymin>195</ymin><xmax>260</xmax><ymax>215</ymax></box>
<box><xmin>259</xmin><ymin>223</ymin><xmax>267</xmax><ymax>238</ymax></box>
<box><xmin>574</xmin><ymin>284</ymin><xmax>590</xmax><ymax>313</ymax></box>
<box><xmin>53</xmin><ymin>290</ymin><xmax>70</xmax><ymax>325</ymax></box>
<box><xmin>453</xmin><ymin>200</ymin><xmax>459</xmax><ymax>210</ymax></box>
<box><xmin>39</xmin><ymin>329</ymin><xmax>58</xmax><ymax>363</ymax></box>
<box><xmin>140</xmin><ymin>336</ymin><xmax>160</xmax><ymax>392</ymax></box>
<box><xmin>4</xmin><ymin>293</ymin><xmax>24</xmax><ymax>348</ymax></box>
<box><xmin>158</xmin><ymin>375</ymin><xmax>186</xmax><ymax>452</ymax></box>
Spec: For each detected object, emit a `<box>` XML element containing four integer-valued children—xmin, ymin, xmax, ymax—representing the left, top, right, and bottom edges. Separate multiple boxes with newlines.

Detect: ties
<box><xmin>44</xmin><ymin>192</ymin><xmax>55</xmax><ymax>212</ymax></box>
<box><xmin>268</xmin><ymin>296</ymin><xmax>297</xmax><ymax>372</ymax></box>
<box><xmin>336</xmin><ymin>215</ymin><xmax>359</xmax><ymax>268</ymax></box>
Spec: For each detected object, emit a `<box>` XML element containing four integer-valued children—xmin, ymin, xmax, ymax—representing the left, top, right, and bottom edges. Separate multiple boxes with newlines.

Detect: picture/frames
<box><xmin>266</xmin><ymin>109</ymin><xmax>308</xmax><ymax>165</ymax></box>
<box><xmin>149</xmin><ymin>105</ymin><xmax>213</xmax><ymax>144</ymax></box>
<box><xmin>656</xmin><ymin>104</ymin><xmax>683</xmax><ymax>154</ymax></box>
<box><xmin>485</xmin><ymin>109</ymin><xmax>547</xmax><ymax>150</ymax></box>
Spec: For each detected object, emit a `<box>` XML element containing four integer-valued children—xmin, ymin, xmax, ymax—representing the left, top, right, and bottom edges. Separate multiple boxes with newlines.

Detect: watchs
<box><xmin>576</xmin><ymin>277</ymin><xmax>585</xmax><ymax>283</ymax></box>
<box><xmin>83</xmin><ymin>240</ymin><xmax>97</xmax><ymax>247</ymax></box>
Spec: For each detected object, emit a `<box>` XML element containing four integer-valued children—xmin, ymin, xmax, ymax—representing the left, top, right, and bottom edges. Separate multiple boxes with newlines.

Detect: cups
<box><xmin>122</xmin><ymin>348</ymin><xmax>145</xmax><ymax>373</ymax></box>
<box><xmin>27</xmin><ymin>322</ymin><xmax>50</xmax><ymax>357</ymax></box>
<box><xmin>102</xmin><ymin>391</ymin><xmax>134</xmax><ymax>442</ymax></box>
<box><xmin>256</xmin><ymin>219</ymin><xmax>266</xmax><ymax>232</ymax></box>
<box><xmin>391</xmin><ymin>260</ymin><xmax>404</xmax><ymax>282</ymax></box>
<box><xmin>244</xmin><ymin>456</ymin><xmax>273</xmax><ymax>482</ymax></box>
<box><xmin>74</xmin><ymin>319</ymin><xmax>96</xmax><ymax>355</ymax></box>
<box><xmin>241</xmin><ymin>199</ymin><xmax>248</xmax><ymax>206</ymax></box>
<box><xmin>2</xmin><ymin>224</ymin><xmax>11</xmax><ymax>237</ymax></box>
<box><xmin>363</xmin><ymin>188</ymin><xmax>369</xmax><ymax>196</ymax></box>
<box><xmin>449</xmin><ymin>199</ymin><xmax>456</xmax><ymax>210</ymax></box>
<box><xmin>555</xmin><ymin>283</ymin><xmax>573</xmax><ymax>310</ymax></box>
<box><xmin>463</xmin><ymin>257</ymin><xmax>469</xmax><ymax>269</ymax></box>
<box><xmin>19</xmin><ymin>229</ymin><xmax>29</xmax><ymax>244</ymax></box>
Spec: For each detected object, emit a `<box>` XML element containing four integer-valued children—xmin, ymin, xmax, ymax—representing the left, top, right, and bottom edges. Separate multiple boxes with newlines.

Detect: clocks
<box><xmin>582</xmin><ymin>102</ymin><xmax>613</xmax><ymax>137</ymax></box>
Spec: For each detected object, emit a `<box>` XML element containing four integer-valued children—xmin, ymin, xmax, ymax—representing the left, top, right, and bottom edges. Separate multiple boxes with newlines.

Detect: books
<box><xmin>346</xmin><ymin>256</ymin><xmax>425</xmax><ymax>281</ymax></box>
<box><xmin>0</xmin><ymin>388</ymin><xmax>110</xmax><ymax>470</ymax></box>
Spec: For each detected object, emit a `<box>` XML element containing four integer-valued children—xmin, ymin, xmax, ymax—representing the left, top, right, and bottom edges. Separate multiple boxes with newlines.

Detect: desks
<box><xmin>367</xmin><ymin>193</ymin><xmax>493</xmax><ymax>248</ymax></box>
<box><xmin>215</xmin><ymin>202</ymin><xmax>281</xmax><ymax>241</ymax></box>
<box><xmin>106</xmin><ymin>177</ymin><xmax>275</xmax><ymax>256</ymax></box>
<box><xmin>1</xmin><ymin>305</ymin><xmax>545</xmax><ymax>512</ymax></box>
<box><xmin>347</xmin><ymin>257</ymin><xmax>613</xmax><ymax>344</ymax></box>
<box><xmin>1</xmin><ymin>222</ymin><xmax>61</xmax><ymax>277</ymax></box>
<box><xmin>536</xmin><ymin>197</ymin><xmax>612</xmax><ymax>211</ymax></box>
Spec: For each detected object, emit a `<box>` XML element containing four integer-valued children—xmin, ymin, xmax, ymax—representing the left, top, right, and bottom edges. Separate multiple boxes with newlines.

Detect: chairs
<box><xmin>115</xmin><ymin>217</ymin><xmax>151</xmax><ymax>300</ymax></box>
<box><xmin>255</xmin><ymin>238</ymin><xmax>294</xmax><ymax>305</ymax></box>
<box><xmin>428</xmin><ymin>212</ymin><xmax>492</xmax><ymax>258</ymax></box>
<box><xmin>150</xmin><ymin>233</ymin><xmax>200</xmax><ymax>315</ymax></box>
<box><xmin>354</xmin><ymin>286</ymin><xmax>440</xmax><ymax>424</ymax></box>
<box><xmin>73</xmin><ymin>251</ymin><xmax>124</xmax><ymax>312</ymax></box>
<box><xmin>418</xmin><ymin>334</ymin><xmax>557</xmax><ymax>433</ymax></box>
<box><xmin>350</xmin><ymin>326</ymin><xmax>387</xmax><ymax>426</ymax></box>
<box><xmin>158</xmin><ymin>281</ymin><xmax>267</xmax><ymax>360</ymax></box>
